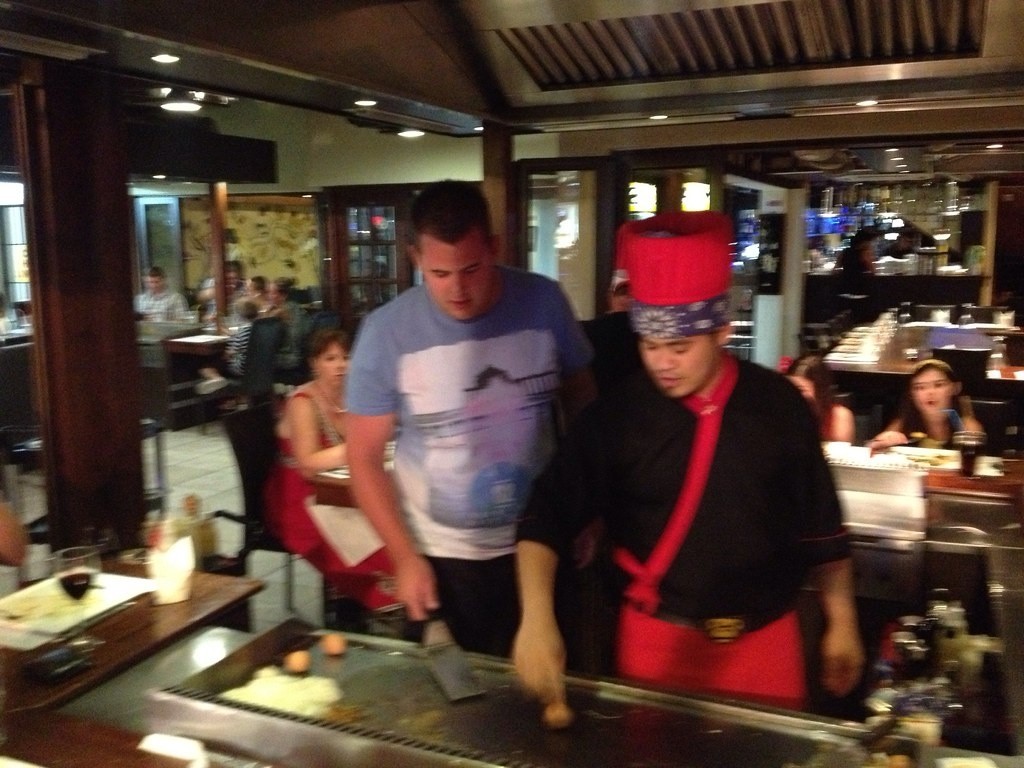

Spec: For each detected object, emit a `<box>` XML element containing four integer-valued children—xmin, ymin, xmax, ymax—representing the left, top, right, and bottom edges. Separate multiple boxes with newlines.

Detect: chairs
<box><xmin>241</xmin><ymin>318</ymin><xmax>286</xmax><ymax>400</ymax></box>
<box><xmin>220</xmin><ymin>404</ymin><xmax>357</xmax><ymax>634</ymax></box>
<box><xmin>311</xmin><ymin>312</ymin><xmax>342</xmax><ymax>331</ymax></box>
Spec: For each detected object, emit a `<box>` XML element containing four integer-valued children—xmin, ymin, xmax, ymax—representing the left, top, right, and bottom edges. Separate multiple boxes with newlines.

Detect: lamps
<box><xmin>932</xmin><ymin>228</ymin><xmax>952</xmax><ymax>241</ymax></box>
<box><xmin>147</xmin><ymin>82</ymin><xmax>230</xmax><ymax>112</ymax></box>
<box><xmin>397</xmin><ymin>125</ymin><xmax>425</xmax><ymax>137</ymax></box>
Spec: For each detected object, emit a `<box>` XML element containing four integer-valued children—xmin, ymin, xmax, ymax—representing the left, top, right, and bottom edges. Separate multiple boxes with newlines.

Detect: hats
<box><xmin>608</xmin><ymin>209</ymin><xmax>735</xmax><ymax>337</ymax></box>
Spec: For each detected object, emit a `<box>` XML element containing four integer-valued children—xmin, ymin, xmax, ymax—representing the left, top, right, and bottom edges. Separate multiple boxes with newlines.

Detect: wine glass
<box><xmin>53</xmin><ymin>545</ymin><xmax>107</xmax><ymax>652</ymax></box>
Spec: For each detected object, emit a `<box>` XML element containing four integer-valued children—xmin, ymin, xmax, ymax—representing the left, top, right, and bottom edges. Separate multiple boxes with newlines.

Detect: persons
<box><xmin>342</xmin><ymin>180</ymin><xmax>588</xmax><ymax>662</ymax></box>
<box><xmin>508</xmin><ymin>207</ymin><xmax>865</xmax><ymax>712</ymax></box>
<box><xmin>605</xmin><ymin>274</ymin><xmax>636</xmax><ymax>312</ymax></box>
<box><xmin>266</xmin><ymin>327</ymin><xmax>408</xmax><ymax>615</ymax></box>
<box><xmin>132</xmin><ymin>262</ymin><xmax>306</xmax><ymax>414</ymax></box>
<box><xmin>866</xmin><ymin>355</ymin><xmax>986</xmax><ymax>454</ymax></box>
<box><xmin>781</xmin><ymin>350</ymin><xmax>857</xmax><ymax>447</ymax></box>
<box><xmin>828</xmin><ymin>229</ymin><xmax>883</xmax><ymax>322</ymax></box>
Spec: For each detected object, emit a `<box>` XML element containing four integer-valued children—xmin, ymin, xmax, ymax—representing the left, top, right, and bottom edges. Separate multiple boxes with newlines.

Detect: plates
<box><xmin>0</xmin><ymin>566</ymin><xmax>155</xmax><ymax>634</ymax></box>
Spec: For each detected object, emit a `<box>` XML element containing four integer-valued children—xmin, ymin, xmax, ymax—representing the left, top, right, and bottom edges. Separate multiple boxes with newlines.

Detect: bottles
<box><xmin>868</xmin><ymin>589</ymin><xmax>987</xmax><ymax>743</ymax></box>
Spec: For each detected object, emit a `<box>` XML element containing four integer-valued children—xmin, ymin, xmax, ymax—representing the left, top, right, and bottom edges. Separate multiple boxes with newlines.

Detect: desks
<box><xmin>0</xmin><ymin>554</ymin><xmax>269</xmax><ymax>717</ymax></box>
<box><xmin>161</xmin><ymin>325</ymin><xmax>246</xmax><ymax>433</ymax></box>
<box><xmin>316</xmin><ymin>437</ymin><xmax>402</xmax><ymax>511</ymax></box>
<box><xmin>137</xmin><ymin>320</ymin><xmax>206</xmax><ymax>404</ymax></box>
